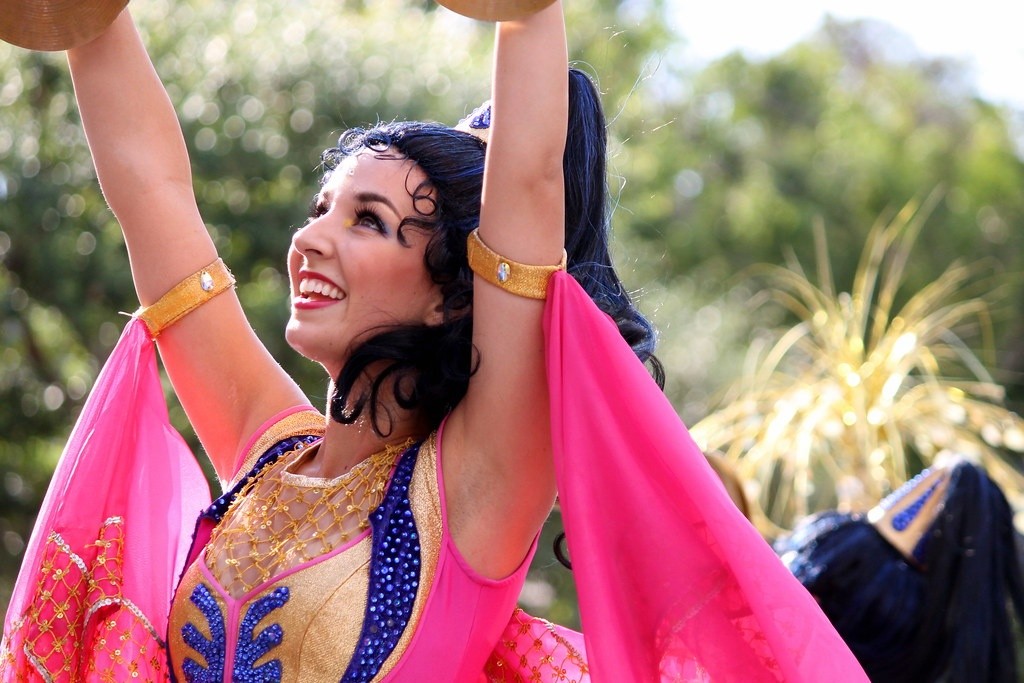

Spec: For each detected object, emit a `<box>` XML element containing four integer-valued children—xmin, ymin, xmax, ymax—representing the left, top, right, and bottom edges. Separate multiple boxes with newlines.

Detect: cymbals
<box><xmin>0</xmin><ymin>0</ymin><xmax>128</xmax><ymax>52</ymax></box>
<box><xmin>434</xmin><ymin>1</ymin><xmax>556</xmax><ymax>24</ymax></box>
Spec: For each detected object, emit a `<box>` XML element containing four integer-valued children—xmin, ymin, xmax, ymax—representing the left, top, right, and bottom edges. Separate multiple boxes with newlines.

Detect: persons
<box><xmin>62</xmin><ymin>0</ymin><xmax>671</xmax><ymax>682</ymax></box>
